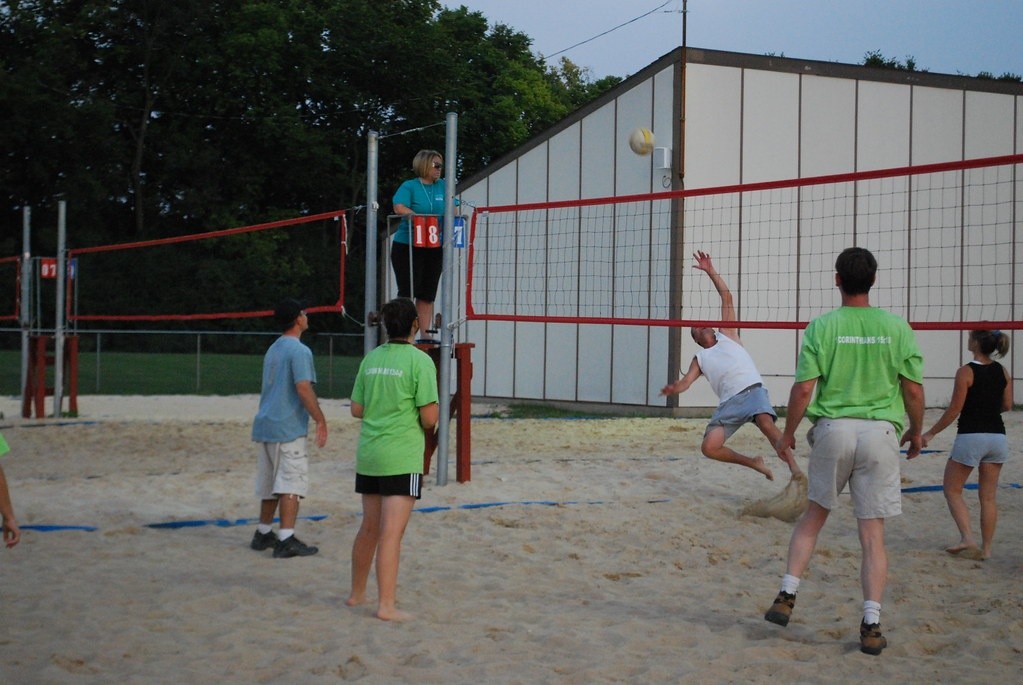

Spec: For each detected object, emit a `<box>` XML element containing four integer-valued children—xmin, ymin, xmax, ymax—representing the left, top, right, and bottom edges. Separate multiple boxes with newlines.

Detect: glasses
<box><xmin>428</xmin><ymin>162</ymin><xmax>443</xmax><ymax>169</ymax></box>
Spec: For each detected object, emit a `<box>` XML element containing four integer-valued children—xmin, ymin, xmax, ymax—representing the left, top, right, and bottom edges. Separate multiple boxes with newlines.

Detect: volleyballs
<box><xmin>628</xmin><ymin>127</ymin><xmax>655</xmax><ymax>156</ymax></box>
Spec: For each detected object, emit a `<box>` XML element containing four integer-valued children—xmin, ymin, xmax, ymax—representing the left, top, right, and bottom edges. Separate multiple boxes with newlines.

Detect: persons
<box><xmin>921</xmin><ymin>320</ymin><xmax>1013</xmax><ymax>561</ymax></box>
<box><xmin>345</xmin><ymin>298</ymin><xmax>440</xmax><ymax>621</ymax></box>
<box><xmin>763</xmin><ymin>247</ymin><xmax>925</xmax><ymax>656</ymax></box>
<box><xmin>660</xmin><ymin>250</ymin><xmax>805</xmax><ymax>482</ymax></box>
<box><xmin>391</xmin><ymin>150</ymin><xmax>458</xmax><ymax>344</ymax></box>
<box><xmin>251</xmin><ymin>299</ymin><xmax>328</xmax><ymax>559</ymax></box>
<box><xmin>0</xmin><ymin>433</ymin><xmax>21</xmax><ymax>549</ymax></box>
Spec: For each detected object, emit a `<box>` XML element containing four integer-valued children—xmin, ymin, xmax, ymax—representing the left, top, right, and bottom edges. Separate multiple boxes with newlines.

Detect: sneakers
<box><xmin>273</xmin><ymin>534</ymin><xmax>319</xmax><ymax>558</ymax></box>
<box><xmin>860</xmin><ymin>616</ymin><xmax>887</xmax><ymax>655</ymax></box>
<box><xmin>764</xmin><ymin>590</ymin><xmax>796</xmax><ymax>627</ymax></box>
<box><xmin>250</xmin><ymin>530</ymin><xmax>279</xmax><ymax>551</ymax></box>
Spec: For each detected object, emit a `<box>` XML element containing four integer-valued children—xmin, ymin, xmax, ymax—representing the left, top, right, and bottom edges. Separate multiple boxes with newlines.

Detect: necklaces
<box><xmin>419</xmin><ymin>177</ymin><xmax>433</xmax><ymax>213</ymax></box>
